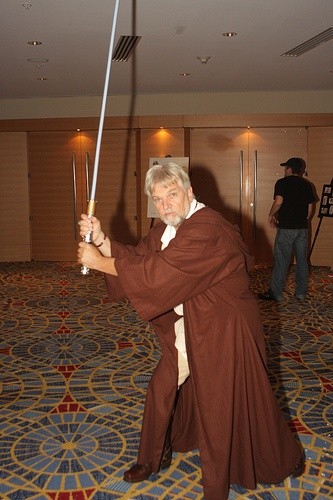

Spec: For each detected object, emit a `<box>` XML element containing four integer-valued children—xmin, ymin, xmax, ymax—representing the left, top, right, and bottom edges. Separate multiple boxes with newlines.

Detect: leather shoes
<box><xmin>123</xmin><ymin>448</ymin><xmax>171</xmax><ymax>482</ymax></box>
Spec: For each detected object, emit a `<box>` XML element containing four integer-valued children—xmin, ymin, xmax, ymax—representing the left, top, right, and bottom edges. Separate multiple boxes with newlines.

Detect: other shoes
<box><xmin>258</xmin><ymin>293</ymin><xmax>275</xmax><ymax>300</ymax></box>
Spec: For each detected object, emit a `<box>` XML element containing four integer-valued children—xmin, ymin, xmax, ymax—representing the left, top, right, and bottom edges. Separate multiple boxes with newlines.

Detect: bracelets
<box><xmin>96</xmin><ymin>233</ymin><xmax>106</xmax><ymax>248</ymax></box>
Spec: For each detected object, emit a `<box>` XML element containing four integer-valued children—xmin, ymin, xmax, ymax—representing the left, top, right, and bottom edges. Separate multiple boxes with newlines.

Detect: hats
<box><xmin>280</xmin><ymin>158</ymin><xmax>306</xmax><ymax>170</ymax></box>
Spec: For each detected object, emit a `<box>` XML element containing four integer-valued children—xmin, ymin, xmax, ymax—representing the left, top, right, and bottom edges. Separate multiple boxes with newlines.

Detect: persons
<box><xmin>77</xmin><ymin>164</ymin><xmax>303</xmax><ymax>500</ymax></box>
<box><xmin>256</xmin><ymin>157</ymin><xmax>320</xmax><ymax>303</ymax></box>
